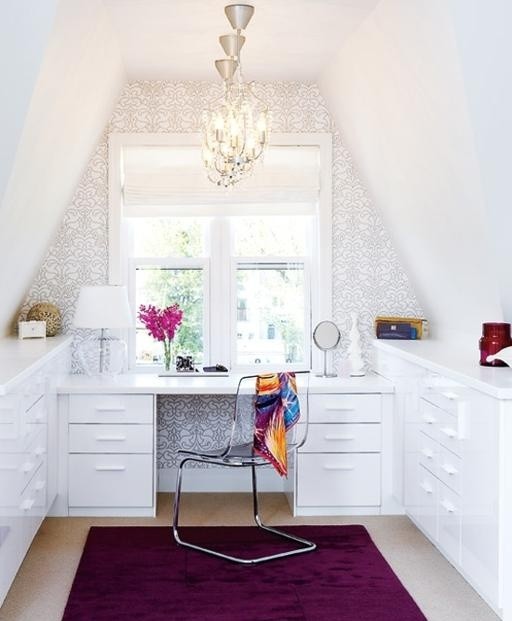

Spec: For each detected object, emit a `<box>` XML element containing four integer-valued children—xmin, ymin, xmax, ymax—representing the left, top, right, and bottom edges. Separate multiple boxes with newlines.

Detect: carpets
<box><xmin>61</xmin><ymin>525</ymin><xmax>429</xmax><ymax>621</ymax></box>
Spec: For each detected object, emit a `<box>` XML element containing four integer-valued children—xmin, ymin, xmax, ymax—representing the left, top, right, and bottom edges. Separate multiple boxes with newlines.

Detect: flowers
<box><xmin>136</xmin><ymin>302</ymin><xmax>184</xmax><ymax>371</ymax></box>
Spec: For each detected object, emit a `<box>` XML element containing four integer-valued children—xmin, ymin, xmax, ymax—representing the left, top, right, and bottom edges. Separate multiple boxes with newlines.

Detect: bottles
<box><xmin>479</xmin><ymin>322</ymin><xmax>512</xmax><ymax>367</ymax></box>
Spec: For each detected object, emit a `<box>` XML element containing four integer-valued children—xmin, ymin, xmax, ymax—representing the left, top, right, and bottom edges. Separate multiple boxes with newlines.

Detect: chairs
<box><xmin>173</xmin><ymin>370</ymin><xmax>317</xmax><ymax>566</ymax></box>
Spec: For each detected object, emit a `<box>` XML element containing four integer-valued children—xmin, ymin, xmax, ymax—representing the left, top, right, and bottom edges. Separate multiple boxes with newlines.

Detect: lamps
<box><xmin>71</xmin><ymin>284</ymin><xmax>134</xmax><ymax>374</ymax></box>
<box><xmin>201</xmin><ymin>4</ymin><xmax>269</xmax><ymax>187</ymax></box>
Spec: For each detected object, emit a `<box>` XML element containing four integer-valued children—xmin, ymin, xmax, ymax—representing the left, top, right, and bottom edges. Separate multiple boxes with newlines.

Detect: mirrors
<box><xmin>313</xmin><ymin>321</ymin><xmax>341</xmax><ymax>378</ymax></box>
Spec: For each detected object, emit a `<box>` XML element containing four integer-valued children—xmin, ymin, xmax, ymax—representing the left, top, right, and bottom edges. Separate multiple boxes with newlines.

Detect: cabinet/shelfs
<box><xmin>0</xmin><ymin>333</ymin><xmax>74</xmax><ymax>610</ymax></box>
<box><xmin>56</xmin><ymin>371</ymin><xmax>396</xmax><ymax>519</ymax></box>
<box><xmin>371</xmin><ymin>339</ymin><xmax>511</xmax><ymax>621</ymax></box>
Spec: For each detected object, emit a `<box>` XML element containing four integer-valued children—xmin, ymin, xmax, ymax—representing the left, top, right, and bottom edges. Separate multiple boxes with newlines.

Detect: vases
<box><xmin>164</xmin><ymin>352</ymin><xmax>174</xmax><ymax>371</ymax></box>
